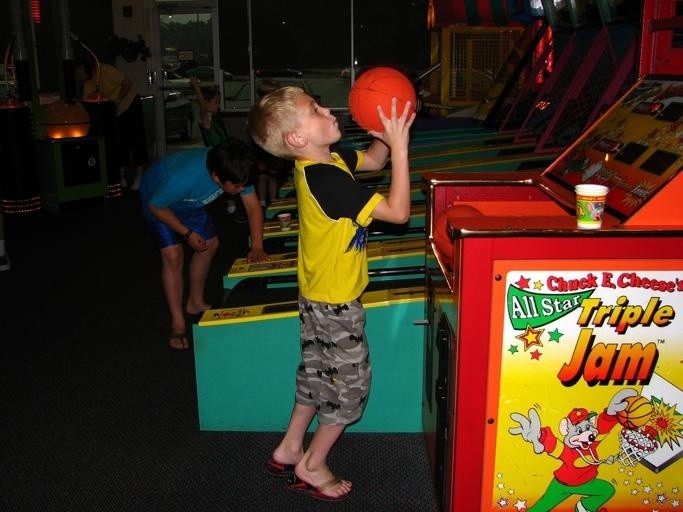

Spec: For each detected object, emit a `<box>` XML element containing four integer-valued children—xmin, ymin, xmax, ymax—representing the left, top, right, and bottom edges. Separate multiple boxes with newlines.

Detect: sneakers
<box><xmin>121</xmin><ymin>178</ymin><xmax>128</xmax><ymax>187</ymax></box>
<box><xmin>224</xmin><ymin>209</ymin><xmax>248</xmax><ymax>224</ymax></box>
<box><xmin>130</xmin><ymin>176</ymin><xmax>142</xmax><ymax>190</ymax></box>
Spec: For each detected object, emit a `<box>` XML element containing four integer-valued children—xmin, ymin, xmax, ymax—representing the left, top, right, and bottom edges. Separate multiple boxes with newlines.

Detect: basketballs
<box><xmin>347</xmin><ymin>68</ymin><xmax>417</xmax><ymax>136</ymax></box>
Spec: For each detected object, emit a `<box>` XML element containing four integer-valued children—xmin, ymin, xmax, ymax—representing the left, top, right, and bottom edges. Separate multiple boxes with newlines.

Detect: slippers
<box><xmin>168</xmin><ymin>333</ymin><xmax>191</xmax><ymax>352</ymax></box>
<box><xmin>264</xmin><ymin>450</ymin><xmax>352</xmax><ymax>502</ymax></box>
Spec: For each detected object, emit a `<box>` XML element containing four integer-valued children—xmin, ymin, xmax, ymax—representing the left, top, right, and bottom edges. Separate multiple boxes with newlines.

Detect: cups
<box><xmin>574</xmin><ymin>184</ymin><xmax>610</xmax><ymax>230</ymax></box>
<box><xmin>277</xmin><ymin>212</ymin><xmax>292</xmax><ymax>232</ymax></box>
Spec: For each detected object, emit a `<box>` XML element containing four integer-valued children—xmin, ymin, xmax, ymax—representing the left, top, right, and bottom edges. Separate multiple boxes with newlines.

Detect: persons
<box><xmin>248</xmin><ymin>79</ymin><xmax>284</xmax><ymax>208</ymax></box>
<box><xmin>81</xmin><ymin>51</ymin><xmax>149</xmax><ymax>192</ymax></box>
<box><xmin>142</xmin><ymin>137</ymin><xmax>269</xmax><ymax>349</ymax></box>
<box><xmin>245</xmin><ymin>86</ymin><xmax>419</xmax><ymax>501</ymax></box>
<box><xmin>189</xmin><ymin>76</ymin><xmax>230</xmax><ymax>145</ymax></box>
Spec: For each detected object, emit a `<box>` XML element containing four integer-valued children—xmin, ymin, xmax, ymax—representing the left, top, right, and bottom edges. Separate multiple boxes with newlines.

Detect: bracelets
<box><xmin>179</xmin><ymin>228</ymin><xmax>192</xmax><ymax>242</ymax></box>
